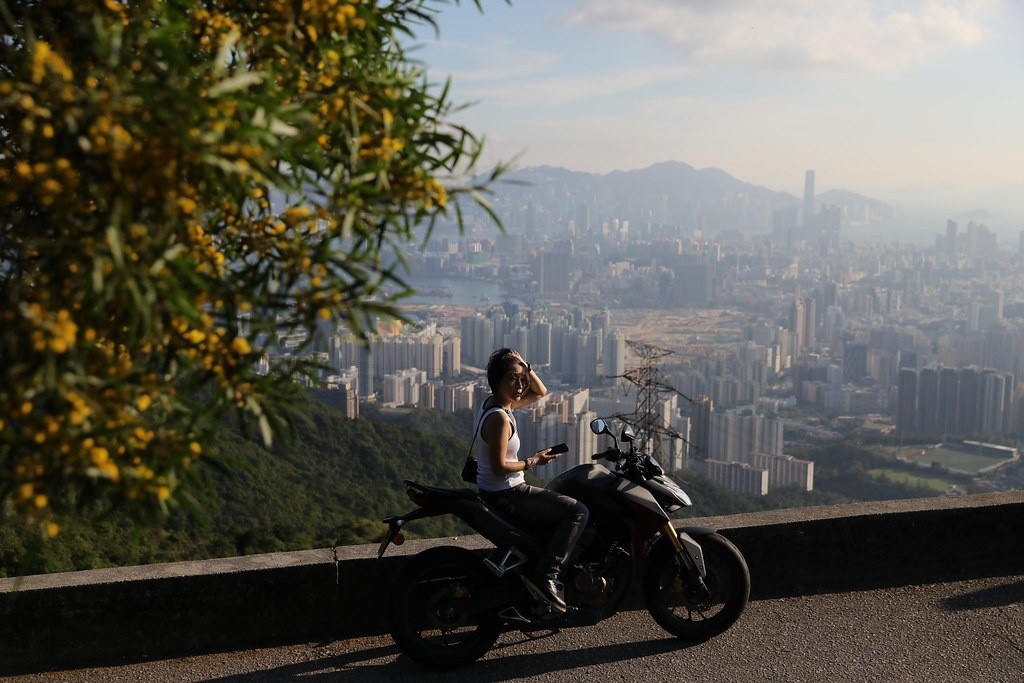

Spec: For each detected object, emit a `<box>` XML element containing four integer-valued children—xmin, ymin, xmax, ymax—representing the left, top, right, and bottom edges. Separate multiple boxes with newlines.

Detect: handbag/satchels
<box><xmin>461</xmin><ymin>454</ymin><xmax>478</xmax><ymax>483</ymax></box>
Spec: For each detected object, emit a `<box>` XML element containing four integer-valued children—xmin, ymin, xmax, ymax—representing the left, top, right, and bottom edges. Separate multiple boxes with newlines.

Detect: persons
<box><xmin>470</xmin><ymin>346</ymin><xmax>591</xmax><ymax>615</ymax></box>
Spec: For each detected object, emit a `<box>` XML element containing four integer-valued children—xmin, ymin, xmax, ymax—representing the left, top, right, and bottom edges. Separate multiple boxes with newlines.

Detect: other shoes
<box><xmin>525</xmin><ymin>572</ymin><xmax>566</xmax><ymax>612</ymax></box>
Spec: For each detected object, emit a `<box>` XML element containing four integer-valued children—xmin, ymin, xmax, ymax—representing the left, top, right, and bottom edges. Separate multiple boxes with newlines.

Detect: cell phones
<box><xmin>537</xmin><ymin>443</ymin><xmax>570</xmax><ymax>456</ymax></box>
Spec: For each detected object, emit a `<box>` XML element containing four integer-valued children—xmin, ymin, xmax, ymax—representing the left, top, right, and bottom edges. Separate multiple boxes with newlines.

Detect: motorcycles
<box><xmin>370</xmin><ymin>417</ymin><xmax>751</xmax><ymax>673</ymax></box>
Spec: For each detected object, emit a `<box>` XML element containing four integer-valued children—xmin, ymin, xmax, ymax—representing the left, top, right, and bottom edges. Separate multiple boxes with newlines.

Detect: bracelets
<box><xmin>526</xmin><ymin>362</ymin><xmax>532</xmax><ymax>374</ymax></box>
<box><xmin>523</xmin><ymin>459</ymin><xmax>530</xmax><ymax>471</ymax></box>
